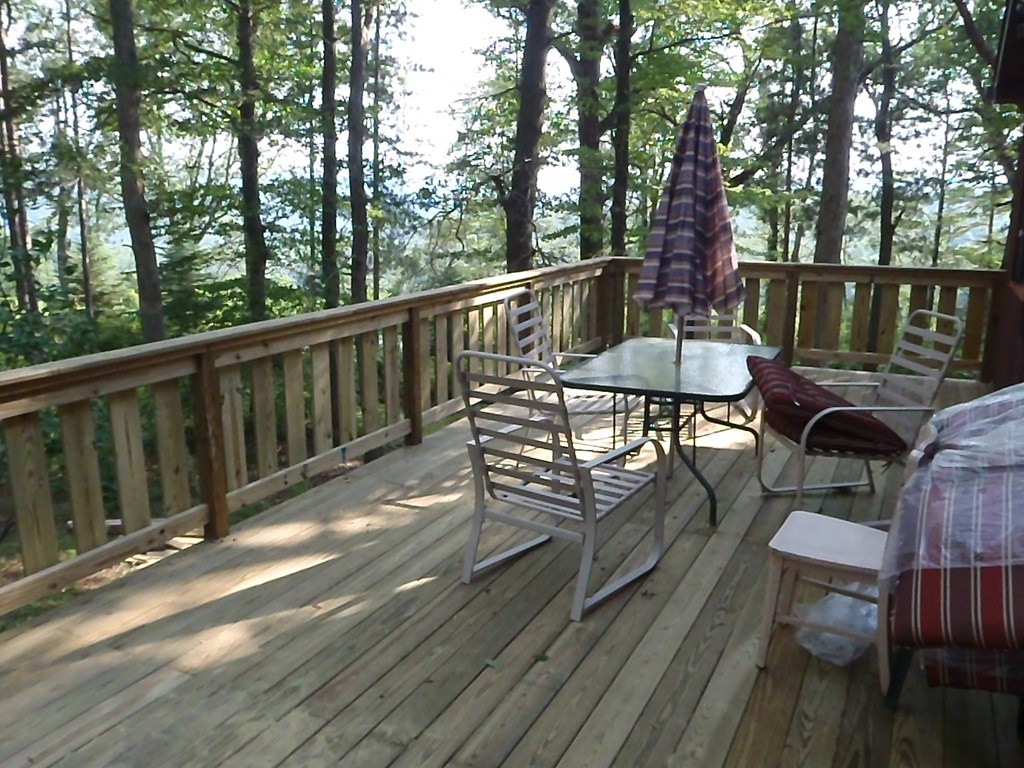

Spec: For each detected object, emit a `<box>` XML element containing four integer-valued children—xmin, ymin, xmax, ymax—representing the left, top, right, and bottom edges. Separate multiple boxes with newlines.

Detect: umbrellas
<box><xmin>631</xmin><ymin>83</ymin><xmax>749</xmax><ymax>479</ymax></box>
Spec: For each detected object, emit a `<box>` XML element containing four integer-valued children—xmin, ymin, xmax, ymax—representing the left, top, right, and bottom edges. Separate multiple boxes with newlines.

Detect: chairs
<box><xmin>503</xmin><ymin>289</ymin><xmax>644</xmax><ymax>469</ymax></box>
<box><xmin>755</xmin><ymin>309</ymin><xmax>963</xmax><ymax>529</ymax></box>
<box><xmin>756</xmin><ymin>423</ymin><xmax>938</xmax><ymax>699</ymax></box>
<box><xmin>665</xmin><ymin>310</ymin><xmax>762</xmax><ymax>424</ymax></box>
<box><xmin>455</xmin><ymin>351</ymin><xmax>668</xmax><ymax>622</ymax></box>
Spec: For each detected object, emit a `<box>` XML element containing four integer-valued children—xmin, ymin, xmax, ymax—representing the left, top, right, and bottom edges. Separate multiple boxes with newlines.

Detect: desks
<box><xmin>560</xmin><ymin>337</ymin><xmax>784</xmax><ymax>527</ymax></box>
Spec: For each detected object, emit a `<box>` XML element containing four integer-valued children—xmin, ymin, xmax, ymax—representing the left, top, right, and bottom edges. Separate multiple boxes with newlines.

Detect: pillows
<box><xmin>745</xmin><ymin>355</ymin><xmax>907</xmax><ymax>454</ymax></box>
